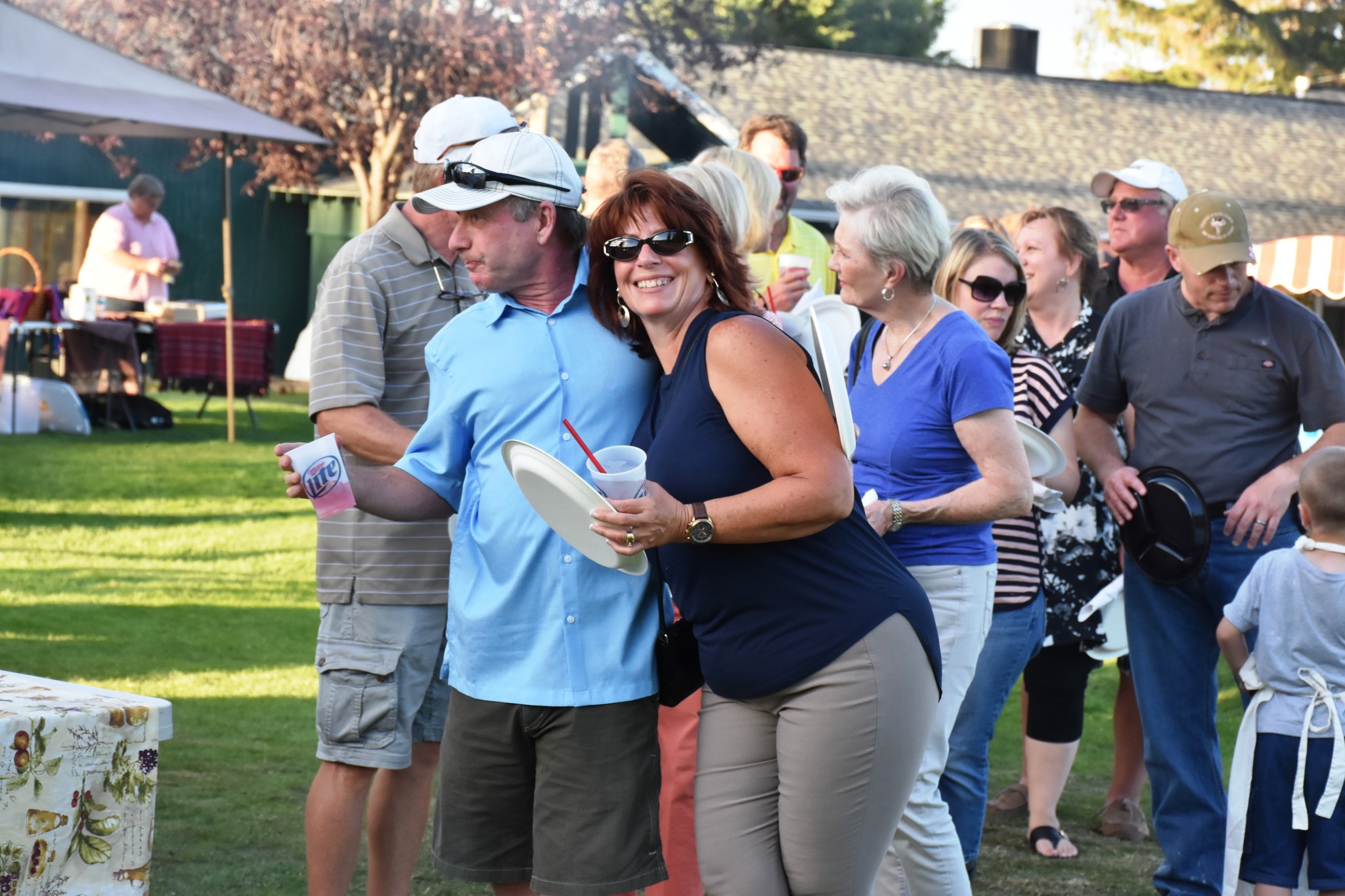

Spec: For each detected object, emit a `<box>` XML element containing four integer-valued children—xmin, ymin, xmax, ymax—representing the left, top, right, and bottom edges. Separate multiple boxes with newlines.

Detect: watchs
<box><xmin>685</xmin><ymin>501</ymin><xmax>714</xmax><ymax>545</ymax></box>
<box><xmin>888</xmin><ymin>497</ymin><xmax>903</xmax><ymax>533</ymax></box>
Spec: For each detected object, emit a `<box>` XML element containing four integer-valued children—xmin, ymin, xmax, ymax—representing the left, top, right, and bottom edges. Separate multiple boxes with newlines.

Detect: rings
<box><xmin>1255</xmin><ymin>520</ymin><xmax>1266</xmax><ymax>527</ymax></box>
<box><xmin>625</xmin><ymin>529</ymin><xmax>634</xmax><ymax>548</ymax></box>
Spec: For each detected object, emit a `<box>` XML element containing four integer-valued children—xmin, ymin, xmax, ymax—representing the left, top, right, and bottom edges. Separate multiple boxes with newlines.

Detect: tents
<box><xmin>1</xmin><ymin>0</ymin><xmax>334</xmax><ymax>449</ymax></box>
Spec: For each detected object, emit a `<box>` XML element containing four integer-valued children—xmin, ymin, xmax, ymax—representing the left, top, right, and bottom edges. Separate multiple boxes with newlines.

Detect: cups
<box><xmin>778</xmin><ymin>254</ymin><xmax>813</xmax><ymax>285</ymax></box>
<box><xmin>284</xmin><ymin>432</ymin><xmax>356</xmax><ymax>520</ymax></box>
<box><xmin>586</xmin><ymin>446</ymin><xmax>648</xmax><ymax>499</ymax></box>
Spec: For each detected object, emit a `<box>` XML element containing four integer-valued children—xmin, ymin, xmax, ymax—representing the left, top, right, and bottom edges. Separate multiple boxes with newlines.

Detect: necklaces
<box><xmin>883</xmin><ymin>292</ymin><xmax>937</xmax><ymax>370</ymax></box>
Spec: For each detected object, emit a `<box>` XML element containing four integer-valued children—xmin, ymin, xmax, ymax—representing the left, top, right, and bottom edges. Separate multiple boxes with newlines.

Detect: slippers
<box><xmin>1028</xmin><ymin>825</ymin><xmax>1079</xmax><ymax>858</ymax></box>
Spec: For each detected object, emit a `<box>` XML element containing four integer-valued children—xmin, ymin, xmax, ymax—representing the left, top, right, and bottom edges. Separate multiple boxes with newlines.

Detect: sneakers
<box><xmin>1100</xmin><ymin>794</ymin><xmax>1150</xmax><ymax>841</ymax></box>
<box><xmin>985</xmin><ymin>784</ymin><xmax>1028</xmax><ymax>820</ymax></box>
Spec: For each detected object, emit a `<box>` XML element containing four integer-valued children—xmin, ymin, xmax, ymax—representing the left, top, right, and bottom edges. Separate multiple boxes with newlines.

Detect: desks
<box><xmin>4</xmin><ymin>313</ymin><xmax>280</xmax><ymax>435</ymax></box>
<box><xmin>0</xmin><ymin>667</ymin><xmax>174</xmax><ymax>896</ymax></box>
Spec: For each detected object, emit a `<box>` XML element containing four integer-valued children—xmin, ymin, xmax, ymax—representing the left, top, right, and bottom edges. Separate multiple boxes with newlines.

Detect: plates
<box><xmin>1016</xmin><ymin>417</ymin><xmax>1069</xmax><ymax>481</ymax></box>
<box><xmin>810</xmin><ymin>308</ymin><xmax>855</xmax><ymax>467</ymax></box>
<box><xmin>501</xmin><ymin>440</ymin><xmax>648</xmax><ymax>575</ymax></box>
<box><xmin>810</xmin><ymin>296</ymin><xmax>863</xmax><ymax>373</ymax></box>
<box><xmin>1119</xmin><ymin>465</ymin><xmax>1210</xmax><ymax>589</ymax></box>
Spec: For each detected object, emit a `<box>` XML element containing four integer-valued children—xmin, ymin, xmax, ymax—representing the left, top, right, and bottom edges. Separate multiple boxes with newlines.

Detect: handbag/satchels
<box><xmin>654</xmin><ymin>618</ymin><xmax>703</xmax><ymax>710</ymax></box>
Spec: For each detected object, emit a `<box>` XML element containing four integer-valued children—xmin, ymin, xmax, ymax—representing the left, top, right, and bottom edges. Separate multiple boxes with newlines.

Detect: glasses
<box><xmin>958</xmin><ymin>275</ymin><xmax>1027</xmax><ymax>307</ymax></box>
<box><xmin>768</xmin><ymin>163</ymin><xmax>805</xmax><ymax>183</ymax></box>
<box><xmin>602</xmin><ymin>228</ymin><xmax>702</xmax><ymax>261</ymax></box>
<box><xmin>413</xmin><ymin>224</ymin><xmax>488</xmax><ymax>301</ymax></box>
<box><xmin>441</xmin><ymin>158</ymin><xmax>571</xmax><ymax>193</ymax></box>
<box><xmin>1100</xmin><ymin>197</ymin><xmax>1170</xmax><ymax>213</ymax></box>
<box><xmin>437</xmin><ymin>122</ymin><xmax>528</xmax><ymax>160</ymax></box>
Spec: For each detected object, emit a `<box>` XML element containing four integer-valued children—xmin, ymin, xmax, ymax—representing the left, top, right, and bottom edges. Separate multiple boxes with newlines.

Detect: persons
<box><xmin>930</xmin><ymin>228</ymin><xmax>1081</xmax><ymax>882</ymax></box>
<box><xmin>302</xmin><ymin>93</ymin><xmax>523</xmax><ymax>895</ymax></box>
<box><xmin>1216</xmin><ymin>443</ymin><xmax>1345</xmax><ymax>896</ymax></box>
<box><xmin>655</xmin><ymin>111</ymin><xmax>839</xmax><ymax>896</ymax></box>
<box><xmin>988</xmin><ymin>156</ymin><xmax>1190</xmax><ymax>842</ymax></box>
<box><xmin>821</xmin><ymin>160</ymin><xmax>1034</xmax><ymax>896</ymax></box>
<box><xmin>1009</xmin><ymin>204</ymin><xmax>1137</xmax><ymax>861</ymax></box>
<box><xmin>1072</xmin><ymin>188</ymin><xmax>1345</xmax><ymax>896</ymax></box>
<box><xmin>582</xmin><ymin>166</ymin><xmax>945</xmax><ymax>896</ymax></box>
<box><xmin>274</xmin><ymin>129</ymin><xmax>669</xmax><ymax>896</ymax></box>
<box><xmin>75</xmin><ymin>171</ymin><xmax>182</xmax><ymax>397</ymax></box>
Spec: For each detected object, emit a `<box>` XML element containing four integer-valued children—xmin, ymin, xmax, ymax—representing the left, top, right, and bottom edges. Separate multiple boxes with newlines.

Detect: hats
<box><xmin>411</xmin><ymin>93</ymin><xmax>520</xmax><ymax>163</ymax></box>
<box><xmin>1090</xmin><ymin>157</ymin><xmax>1188</xmax><ymax>203</ymax></box>
<box><xmin>411</xmin><ymin>128</ymin><xmax>584</xmax><ymax>214</ymax></box>
<box><xmin>1167</xmin><ymin>188</ymin><xmax>1256</xmax><ymax>276</ymax></box>
